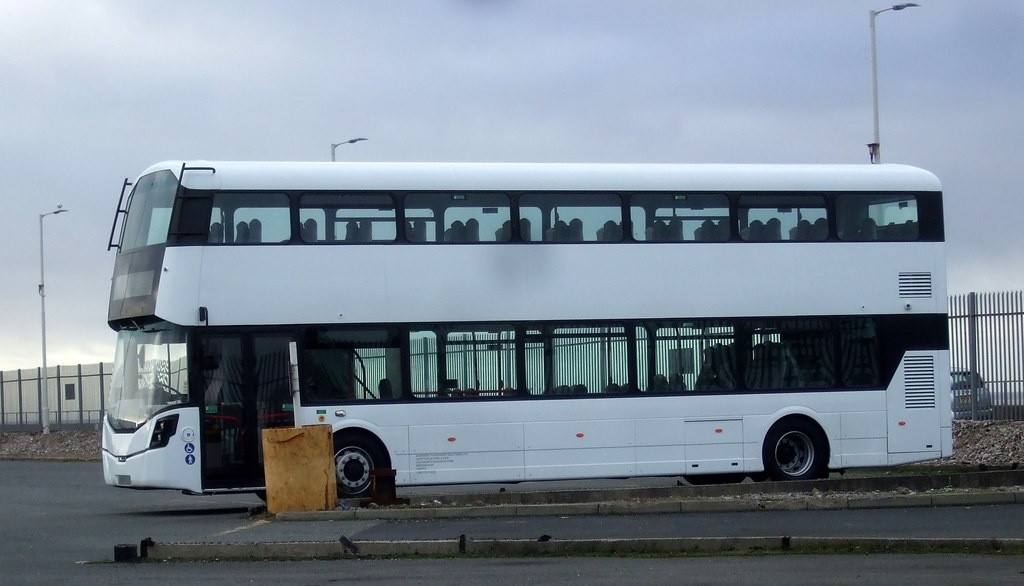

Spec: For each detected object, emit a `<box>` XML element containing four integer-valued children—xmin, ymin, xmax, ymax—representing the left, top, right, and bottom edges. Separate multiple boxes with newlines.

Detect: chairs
<box><xmin>205</xmin><ymin>217</ymin><xmax>920</xmax><ymax>397</ymax></box>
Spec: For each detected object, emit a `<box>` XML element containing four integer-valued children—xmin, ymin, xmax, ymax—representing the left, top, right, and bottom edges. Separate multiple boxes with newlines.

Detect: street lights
<box><xmin>868</xmin><ymin>2</ymin><xmax>921</xmax><ymax>165</ymax></box>
<box><xmin>39</xmin><ymin>210</ymin><xmax>69</xmax><ymax>434</ymax></box>
<box><xmin>331</xmin><ymin>138</ymin><xmax>369</xmax><ymax>162</ymax></box>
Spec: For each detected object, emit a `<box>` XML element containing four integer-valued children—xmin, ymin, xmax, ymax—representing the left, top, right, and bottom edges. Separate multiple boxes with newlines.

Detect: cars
<box><xmin>949</xmin><ymin>367</ymin><xmax>993</xmax><ymax>419</ymax></box>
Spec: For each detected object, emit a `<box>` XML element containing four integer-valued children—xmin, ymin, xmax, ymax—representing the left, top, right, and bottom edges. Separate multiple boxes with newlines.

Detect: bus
<box><xmin>101</xmin><ymin>160</ymin><xmax>955</xmax><ymax>502</ymax></box>
